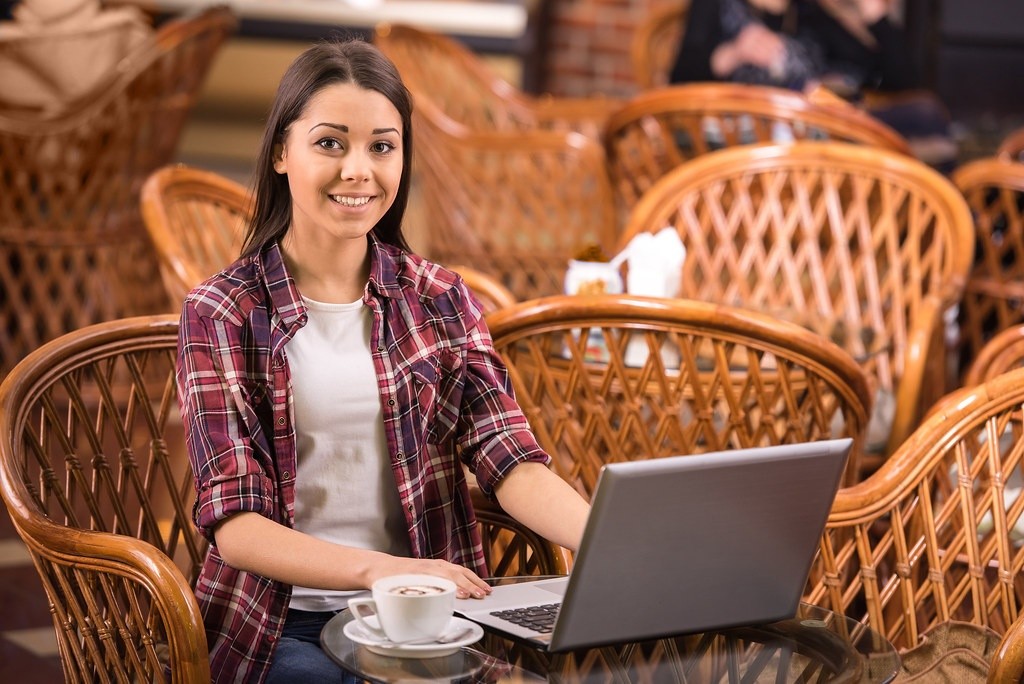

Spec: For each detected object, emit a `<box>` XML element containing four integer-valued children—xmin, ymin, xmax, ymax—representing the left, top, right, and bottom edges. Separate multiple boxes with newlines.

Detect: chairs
<box><xmin>0</xmin><ymin>0</ymin><xmax>1024</xmax><ymax>683</ymax></box>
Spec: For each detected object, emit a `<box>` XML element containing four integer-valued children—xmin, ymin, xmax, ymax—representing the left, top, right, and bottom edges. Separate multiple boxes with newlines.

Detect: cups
<box><xmin>349</xmin><ymin>575</ymin><xmax>456</xmax><ymax>644</ymax></box>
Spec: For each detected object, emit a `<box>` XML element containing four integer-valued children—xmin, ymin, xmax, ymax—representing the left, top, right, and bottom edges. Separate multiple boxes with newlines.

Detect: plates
<box><xmin>343</xmin><ymin>615</ymin><xmax>484</xmax><ymax>659</ymax></box>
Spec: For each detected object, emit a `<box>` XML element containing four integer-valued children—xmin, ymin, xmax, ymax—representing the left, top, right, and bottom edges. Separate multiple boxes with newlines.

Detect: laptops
<box><xmin>453</xmin><ymin>438</ymin><xmax>855</xmax><ymax>651</ymax></box>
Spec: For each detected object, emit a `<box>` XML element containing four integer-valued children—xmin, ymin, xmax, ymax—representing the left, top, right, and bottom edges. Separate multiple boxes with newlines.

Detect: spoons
<box><xmin>377</xmin><ymin>628</ymin><xmax>474</xmax><ymax>647</ymax></box>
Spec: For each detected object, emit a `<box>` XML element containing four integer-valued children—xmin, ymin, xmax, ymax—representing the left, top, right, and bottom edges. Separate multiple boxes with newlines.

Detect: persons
<box><xmin>669</xmin><ymin>1</ymin><xmax>964</xmax><ymax>178</ymax></box>
<box><xmin>174</xmin><ymin>41</ymin><xmax>594</xmax><ymax>683</ymax></box>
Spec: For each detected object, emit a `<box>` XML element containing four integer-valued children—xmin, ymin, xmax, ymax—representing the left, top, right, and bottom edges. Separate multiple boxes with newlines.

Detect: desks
<box><xmin>519</xmin><ymin>302</ymin><xmax>888</xmax><ymax>399</ymax></box>
<box><xmin>348</xmin><ymin>576</ymin><xmax>901</xmax><ymax>683</ymax></box>
